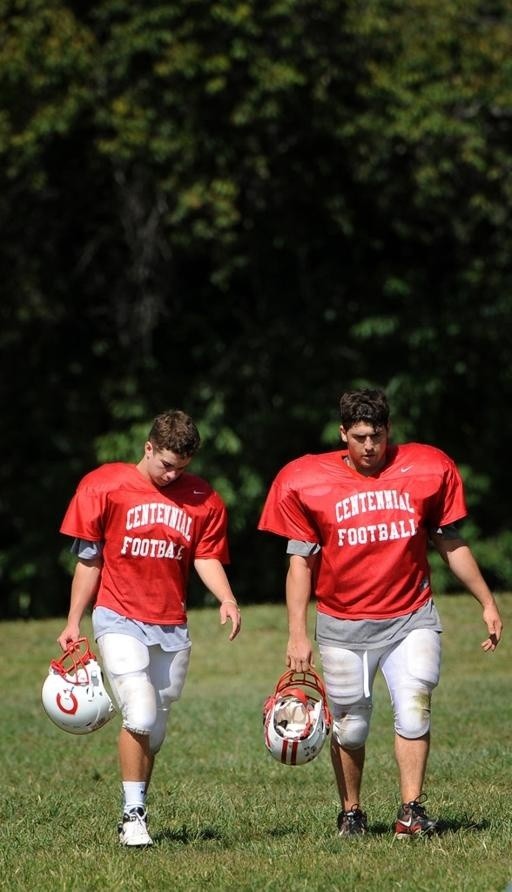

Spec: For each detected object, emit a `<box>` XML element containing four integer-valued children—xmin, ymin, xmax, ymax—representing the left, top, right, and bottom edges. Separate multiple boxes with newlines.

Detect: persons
<box><xmin>39</xmin><ymin>407</ymin><xmax>241</xmax><ymax>849</ymax></box>
<box><xmin>256</xmin><ymin>393</ymin><xmax>509</xmax><ymax>841</ymax></box>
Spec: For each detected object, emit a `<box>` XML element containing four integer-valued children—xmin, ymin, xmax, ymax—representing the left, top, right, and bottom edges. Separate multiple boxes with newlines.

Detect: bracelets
<box><xmin>222</xmin><ymin>600</ymin><xmax>240</xmax><ymax>612</ymax></box>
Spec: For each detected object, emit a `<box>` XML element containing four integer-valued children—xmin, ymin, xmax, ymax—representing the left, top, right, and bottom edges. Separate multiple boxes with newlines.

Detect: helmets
<box><xmin>39</xmin><ymin>635</ymin><xmax>119</xmax><ymax>737</ymax></box>
<box><xmin>262</xmin><ymin>665</ymin><xmax>333</xmax><ymax>767</ymax></box>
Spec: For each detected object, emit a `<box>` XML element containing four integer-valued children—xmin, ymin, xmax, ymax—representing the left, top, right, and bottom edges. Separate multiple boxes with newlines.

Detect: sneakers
<box><xmin>337</xmin><ymin>803</ymin><xmax>370</xmax><ymax>839</ymax></box>
<box><xmin>123</xmin><ymin>801</ymin><xmax>155</xmax><ymax>849</ymax></box>
<box><xmin>395</xmin><ymin>800</ymin><xmax>448</xmax><ymax>841</ymax></box>
